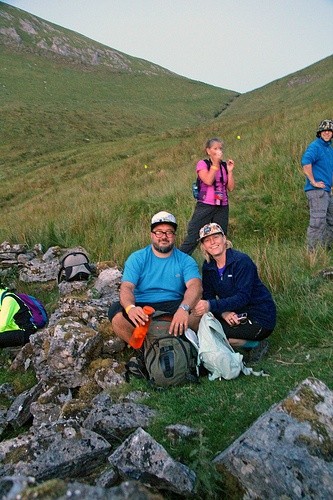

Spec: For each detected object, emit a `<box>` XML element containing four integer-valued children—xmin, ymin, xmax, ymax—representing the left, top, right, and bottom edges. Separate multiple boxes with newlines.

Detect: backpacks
<box><xmin>144</xmin><ymin>311</ymin><xmax>200</xmax><ymax>388</ymax></box>
<box><xmin>2</xmin><ymin>290</ymin><xmax>47</xmax><ymax>328</ymax></box>
<box><xmin>58</xmin><ymin>252</ymin><xmax>92</xmax><ymax>283</ymax></box>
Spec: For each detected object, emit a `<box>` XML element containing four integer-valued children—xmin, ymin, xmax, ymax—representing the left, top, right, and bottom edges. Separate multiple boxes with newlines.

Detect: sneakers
<box><xmin>248</xmin><ymin>341</ymin><xmax>269</xmax><ymax>361</ymax></box>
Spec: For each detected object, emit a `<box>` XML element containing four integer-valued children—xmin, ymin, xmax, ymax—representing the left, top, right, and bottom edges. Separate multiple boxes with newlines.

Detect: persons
<box><xmin>0</xmin><ymin>287</ymin><xmax>37</xmax><ymax>348</ymax></box>
<box><xmin>178</xmin><ymin>138</ymin><xmax>235</xmax><ymax>258</ymax></box>
<box><xmin>112</xmin><ymin>211</ymin><xmax>203</xmax><ymax>353</ymax></box>
<box><xmin>301</xmin><ymin>119</ymin><xmax>333</xmax><ymax>279</ymax></box>
<box><xmin>191</xmin><ymin>223</ymin><xmax>278</xmax><ymax>367</ymax></box>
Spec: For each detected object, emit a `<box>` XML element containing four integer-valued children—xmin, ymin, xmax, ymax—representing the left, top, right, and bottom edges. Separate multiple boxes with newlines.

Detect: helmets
<box><xmin>317</xmin><ymin>120</ymin><xmax>333</xmax><ymax>132</ymax></box>
<box><xmin>151</xmin><ymin>211</ymin><xmax>178</xmax><ymax>231</ymax></box>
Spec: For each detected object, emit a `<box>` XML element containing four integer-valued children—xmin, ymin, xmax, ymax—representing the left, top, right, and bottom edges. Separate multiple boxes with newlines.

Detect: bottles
<box><xmin>128</xmin><ymin>305</ymin><xmax>155</xmax><ymax>349</ymax></box>
<box><xmin>192</xmin><ymin>182</ymin><xmax>199</xmax><ymax>198</ymax></box>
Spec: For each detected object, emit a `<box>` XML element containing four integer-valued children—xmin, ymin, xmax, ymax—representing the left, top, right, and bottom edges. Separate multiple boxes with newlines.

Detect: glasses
<box><xmin>151</xmin><ymin>230</ymin><xmax>176</xmax><ymax>238</ymax></box>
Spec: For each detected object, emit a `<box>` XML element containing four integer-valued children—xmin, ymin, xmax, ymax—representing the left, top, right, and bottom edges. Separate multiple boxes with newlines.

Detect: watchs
<box><xmin>179</xmin><ymin>303</ymin><xmax>190</xmax><ymax>312</ymax></box>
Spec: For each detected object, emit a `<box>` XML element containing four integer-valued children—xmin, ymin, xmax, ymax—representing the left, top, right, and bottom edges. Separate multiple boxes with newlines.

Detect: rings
<box><xmin>228</xmin><ymin>319</ymin><xmax>232</xmax><ymax>321</ymax></box>
<box><xmin>233</xmin><ymin>314</ymin><xmax>237</xmax><ymax>316</ymax></box>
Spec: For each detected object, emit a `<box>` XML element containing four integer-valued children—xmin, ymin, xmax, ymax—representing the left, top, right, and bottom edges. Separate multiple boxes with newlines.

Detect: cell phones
<box><xmin>236</xmin><ymin>313</ymin><xmax>248</xmax><ymax>320</ymax></box>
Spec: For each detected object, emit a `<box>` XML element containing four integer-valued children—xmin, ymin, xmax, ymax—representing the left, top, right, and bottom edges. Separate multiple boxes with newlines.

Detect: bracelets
<box><xmin>210</xmin><ymin>165</ymin><xmax>219</xmax><ymax>171</ymax></box>
<box><xmin>125</xmin><ymin>305</ymin><xmax>136</xmax><ymax>314</ymax></box>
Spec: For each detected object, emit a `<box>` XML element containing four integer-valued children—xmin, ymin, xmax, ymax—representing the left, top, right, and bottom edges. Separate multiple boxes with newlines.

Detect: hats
<box><xmin>197</xmin><ymin>223</ymin><xmax>224</xmax><ymax>242</ymax></box>
